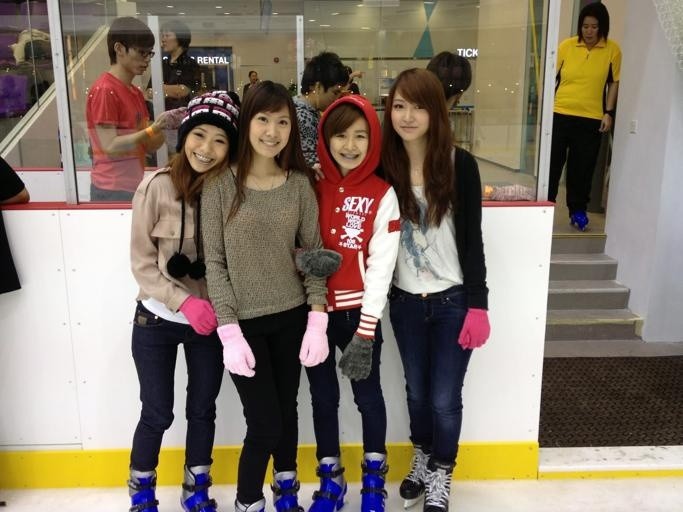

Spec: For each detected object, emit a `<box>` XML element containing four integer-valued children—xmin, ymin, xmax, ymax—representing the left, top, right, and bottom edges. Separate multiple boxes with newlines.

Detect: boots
<box><xmin>360</xmin><ymin>452</ymin><xmax>389</xmax><ymax>511</ymax></box>
<box><xmin>181</xmin><ymin>465</ymin><xmax>218</xmax><ymax>512</ymax></box>
<box><xmin>271</xmin><ymin>469</ymin><xmax>304</xmax><ymax>511</ymax></box>
<box><xmin>400</xmin><ymin>437</ymin><xmax>456</xmax><ymax>512</ymax></box>
<box><xmin>235</xmin><ymin>500</ymin><xmax>266</xmax><ymax>512</ymax></box>
<box><xmin>308</xmin><ymin>455</ymin><xmax>347</xmax><ymax>512</ymax></box>
<box><xmin>128</xmin><ymin>470</ymin><xmax>159</xmax><ymax>512</ymax></box>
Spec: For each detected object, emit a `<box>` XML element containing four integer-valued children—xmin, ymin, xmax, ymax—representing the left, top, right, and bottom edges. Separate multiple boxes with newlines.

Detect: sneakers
<box><xmin>573</xmin><ymin>215</ymin><xmax>589</xmax><ymax>226</ymax></box>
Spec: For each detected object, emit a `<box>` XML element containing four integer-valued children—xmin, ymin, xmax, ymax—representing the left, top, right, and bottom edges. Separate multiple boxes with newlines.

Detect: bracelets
<box><xmin>604</xmin><ymin>109</ymin><xmax>615</xmax><ymax>118</ymax></box>
<box><xmin>165</xmin><ymin>85</ymin><xmax>168</xmax><ymax>97</ymax></box>
<box><xmin>147</xmin><ymin>127</ymin><xmax>155</xmax><ymax>138</ymax></box>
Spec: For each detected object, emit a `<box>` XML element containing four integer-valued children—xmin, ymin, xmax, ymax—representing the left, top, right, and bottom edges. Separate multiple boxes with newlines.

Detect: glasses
<box><xmin>134</xmin><ymin>47</ymin><xmax>154</xmax><ymax>60</ymax></box>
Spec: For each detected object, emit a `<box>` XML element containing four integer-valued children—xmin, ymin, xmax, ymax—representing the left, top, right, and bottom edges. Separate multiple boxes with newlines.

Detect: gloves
<box><xmin>459</xmin><ymin>309</ymin><xmax>490</xmax><ymax>350</ymax></box>
<box><xmin>339</xmin><ymin>335</ymin><xmax>374</xmax><ymax>381</ymax></box>
<box><xmin>299</xmin><ymin>311</ymin><xmax>330</xmax><ymax>367</ymax></box>
<box><xmin>299</xmin><ymin>248</ymin><xmax>345</xmax><ymax>278</ymax></box>
<box><xmin>180</xmin><ymin>296</ymin><xmax>218</xmax><ymax>336</ymax></box>
<box><xmin>214</xmin><ymin>324</ymin><xmax>256</xmax><ymax>377</ymax></box>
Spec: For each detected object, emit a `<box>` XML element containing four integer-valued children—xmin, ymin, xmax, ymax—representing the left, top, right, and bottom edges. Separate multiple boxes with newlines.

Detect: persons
<box><xmin>197</xmin><ymin>80</ymin><xmax>330</xmax><ymax>510</ymax></box>
<box><xmin>426</xmin><ymin>51</ymin><xmax>472</xmax><ymax>111</ymax></box>
<box><xmin>0</xmin><ymin>156</ymin><xmax>31</xmax><ymax>294</ymax></box>
<box><xmin>547</xmin><ymin>2</ymin><xmax>622</xmax><ymax>232</ymax></box>
<box><xmin>145</xmin><ymin>21</ymin><xmax>199</xmax><ymax>166</ymax></box>
<box><xmin>309</xmin><ymin>68</ymin><xmax>492</xmax><ymax>512</ymax></box>
<box><xmin>291</xmin><ymin>52</ymin><xmax>366</xmax><ymax>180</ymax></box>
<box><xmin>124</xmin><ymin>89</ymin><xmax>242</xmax><ymax>510</ymax></box>
<box><xmin>292</xmin><ymin>93</ymin><xmax>402</xmax><ymax>511</ymax></box>
<box><xmin>87</xmin><ymin>17</ymin><xmax>187</xmax><ymax>201</ymax></box>
<box><xmin>243</xmin><ymin>71</ymin><xmax>258</xmax><ymax>94</ymax></box>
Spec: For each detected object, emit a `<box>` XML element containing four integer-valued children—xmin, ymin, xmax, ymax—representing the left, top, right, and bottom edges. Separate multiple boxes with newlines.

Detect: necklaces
<box><xmin>247</xmin><ymin>164</ymin><xmax>279</xmax><ymax>192</ymax></box>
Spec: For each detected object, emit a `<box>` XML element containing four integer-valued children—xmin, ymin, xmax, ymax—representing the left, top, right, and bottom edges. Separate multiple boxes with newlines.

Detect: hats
<box><xmin>177</xmin><ymin>91</ymin><xmax>241</xmax><ymax>164</ymax></box>
